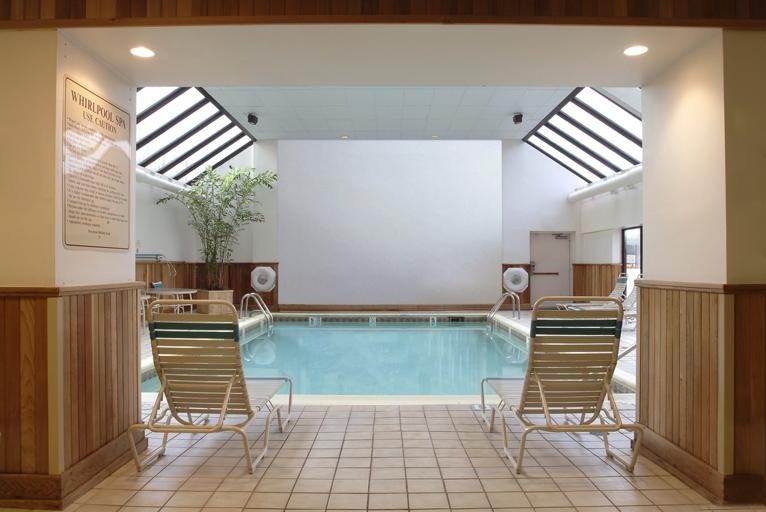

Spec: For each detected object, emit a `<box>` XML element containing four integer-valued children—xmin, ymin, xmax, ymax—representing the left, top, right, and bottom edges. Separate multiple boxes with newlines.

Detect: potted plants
<box><xmin>156</xmin><ymin>164</ymin><xmax>278</xmax><ymax>315</ymax></box>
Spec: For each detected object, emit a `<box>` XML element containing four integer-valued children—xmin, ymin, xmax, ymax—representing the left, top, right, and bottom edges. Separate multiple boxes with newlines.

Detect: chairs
<box><xmin>557</xmin><ymin>270</ymin><xmax>640</xmax><ymax>333</ymax></box>
<box><xmin>126</xmin><ymin>296</ymin><xmax>294</xmax><ymax>475</ymax></box>
<box><xmin>480</xmin><ymin>294</ymin><xmax>640</xmax><ymax>477</ymax></box>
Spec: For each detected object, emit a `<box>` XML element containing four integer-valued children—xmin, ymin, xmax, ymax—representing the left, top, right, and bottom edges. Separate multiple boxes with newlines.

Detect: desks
<box><xmin>146</xmin><ymin>284</ymin><xmax>198</xmax><ymax>320</ymax></box>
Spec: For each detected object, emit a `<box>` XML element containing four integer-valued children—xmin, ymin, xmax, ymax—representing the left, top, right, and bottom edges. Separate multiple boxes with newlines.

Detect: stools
<box><xmin>135</xmin><ymin>294</ymin><xmax>155</xmax><ymax>330</ymax></box>
